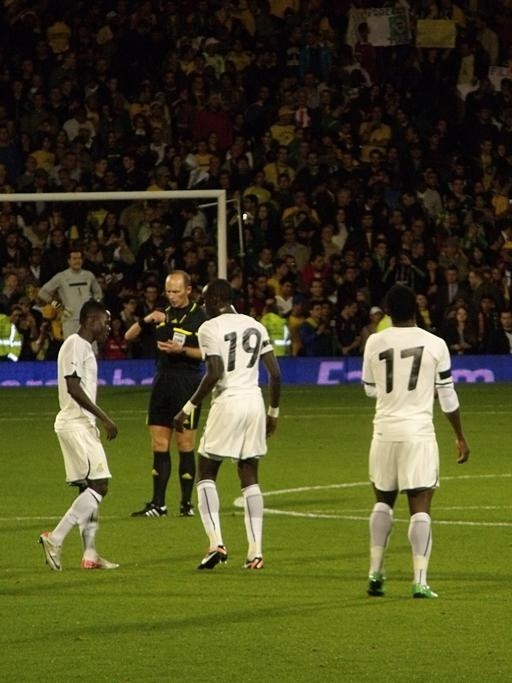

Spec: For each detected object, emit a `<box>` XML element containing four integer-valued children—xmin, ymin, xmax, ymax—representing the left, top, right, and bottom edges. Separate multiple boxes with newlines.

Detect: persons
<box><xmin>446</xmin><ymin>304</ymin><xmax>477</xmax><ymax>355</ymax></box>
<box><xmin>173</xmin><ymin>278</ymin><xmax>283</xmax><ymax>571</ymax></box>
<box><xmin>227</xmin><ymin>0</ymin><xmax>512</xmax><ymax>305</ymax></box>
<box><xmin>38</xmin><ymin>298</ymin><xmax>121</xmax><ymax>572</ymax></box>
<box><xmin>321</xmin><ymin>302</ymin><xmax>331</xmax><ymax>327</ymax></box>
<box><xmin>124</xmin><ymin>270</ymin><xmax>208</xmax><ymax>519</ymax></box>
<box><xmin>360</xmin><ymin>281</ymin><xmax>470</xmax><ymax>600</ymax></box>
<box><xmin>287</xmin><ymin>300</ymin><xmax>306</xmax><ymax>356</ymax></box>
<box><xmin>471</xmin><ymin>296</ymin><xmax>495</xmax><ymax>355</ymax></box>
<box><xmin>0</xmin><ymin>280</ymin><xmax>161</xmax><ymax>366</ymax></box>
<box><xmin>487</xmin><ymin>309</ymin><xmax>512</xmax><ymax>355</ymax></box>
<box><xmin>360</xmin><ymin>306</ymin><xmax>383</xmax><ymax>356</ymax></box>
<box><xmin>299</xmin><ymin>302</ymin><xmax>329</xmax><ymax>357</ymax></box>
<box><xmin>415</xmin><ymin>291</ymin><xmax>445</xmax><ymax>338</ymax></box>
<box><xmin>0</xmin><ymin>0</ymin><xmax>227</xmax><ymax>280</ymax></box>
<box><xmin>329</xmin><ymin>301</ymin><xmax>360</xmax><ymax>357</ymax></box>
<box><xmin>260</xmin><ymin>298</ymin><xmax>292</xmax><ymax>357</ymax></box>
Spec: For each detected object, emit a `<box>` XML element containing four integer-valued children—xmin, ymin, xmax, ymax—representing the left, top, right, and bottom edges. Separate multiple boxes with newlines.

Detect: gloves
<box><xmin>49</xmin><ymin>300</ymin><xmax>72</xmax><ymax>320</ymax></box>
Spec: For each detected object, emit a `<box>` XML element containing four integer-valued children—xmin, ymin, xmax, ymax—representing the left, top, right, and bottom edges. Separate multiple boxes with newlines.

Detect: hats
<box><xmin>204</xmin><ymin>36</ymin><xmax>221</xmax><ymax>50</ymax></box>
<box><xmin>368</xmin><ymin>307</ymin><xmax>384</xmax><ymax>318</ymax></box>
<box><xmin>277</xmin><ymin>105</ymin><xmax>295</xmax><ymax>118</ymax></box>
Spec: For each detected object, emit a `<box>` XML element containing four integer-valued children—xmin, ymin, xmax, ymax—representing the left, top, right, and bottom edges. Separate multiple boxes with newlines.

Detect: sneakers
<box><xmin>35</xmin><ymin>530</ymin><xmax>63</xmax><ymax>572</ymax></box>
<box><xmin>130</xmin><ymin>503</ymin><xmax>168</xmax><ymax>519</ymax></box>
<box><xmin>366</xmin><ymin>571</ymin><xmax>388</xmax><ymax>597</ymax></box>
<box><xmin>241</xmin><ymin>555</ymin><xmax>265</xmax><ymax>570</ymax></box>
<box><xmin>178</xmin><ymin>501</ymin><xmax>195</xmax><ymax>517</ymax></box>
<box><xmin>412</xmin><ymin>584</ymin><xmax>439</xmax><ymax>599</ymax></box>
<box><xmin>197</xmin><ymin>547</ymin><xmax>226</xmax><ymax>569</ymax></box>
<box><xmin>78</xmin><ymin>555</ymin><xmax>121</xmax><ymax>571</ymax></box>
